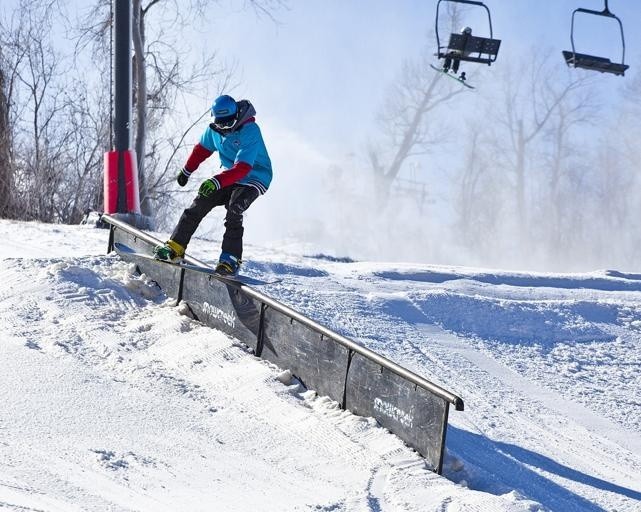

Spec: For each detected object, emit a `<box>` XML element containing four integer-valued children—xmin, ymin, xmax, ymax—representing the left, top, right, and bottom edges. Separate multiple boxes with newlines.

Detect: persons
<box><xmin>442</xmin><ymin>26</ymin><xmax>474</xmax><ymax>71</ymax></box>
<box><xmin>154</xmin><ymin>94</ymin><xmax>273</xmax><ymax>277</ymax></box>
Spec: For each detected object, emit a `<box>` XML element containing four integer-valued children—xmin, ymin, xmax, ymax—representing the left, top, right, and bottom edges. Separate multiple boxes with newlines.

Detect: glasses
<box><xmin>216</xmin><ymin>121</ymin><xmax>237</xmax><ymax>128</ymax></box>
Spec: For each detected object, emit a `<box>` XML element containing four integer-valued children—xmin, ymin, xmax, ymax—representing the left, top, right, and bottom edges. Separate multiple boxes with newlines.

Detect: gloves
<box><xmin>198</xmin><ymin>177</ymin><xmax>219</xmax><ymax>198</ymax></box>
<box><xmin>177</xmin><ymin>169</ymin><xmax>191</xmax><ymax>186</ymax></box>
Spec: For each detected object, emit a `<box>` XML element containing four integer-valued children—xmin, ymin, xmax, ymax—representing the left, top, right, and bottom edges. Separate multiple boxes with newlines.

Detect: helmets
<box><xmin>213</xmin><ymin>95</ymin><xmax>237</xmax><ymax>124</ymax></box>
<box><xmin>461</xmin><ymin>27</ymin><xmax>472</xmax><ymax>35</ymax></box>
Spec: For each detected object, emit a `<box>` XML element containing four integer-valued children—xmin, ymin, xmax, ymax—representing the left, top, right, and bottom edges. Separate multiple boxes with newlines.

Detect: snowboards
<box><xmin>429</xmin><ymin>63</ymin><xmax>478</xmax><ymax>90</ymax></box>
<box><xmin>113</xmin><ymin>242</ymin><xmax>283</xmax><ymax>285</ymax></box>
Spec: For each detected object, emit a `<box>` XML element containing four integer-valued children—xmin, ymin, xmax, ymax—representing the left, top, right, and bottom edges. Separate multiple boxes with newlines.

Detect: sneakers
<box><xmin>156</xmin><ymin>247</ymin><xmax>184</xmax><ymax>260</ymax></box>
<box><xmin>216</xmin><ymin>262</ymin><xmax>234</xmax><ymax>274</ymax></box>
